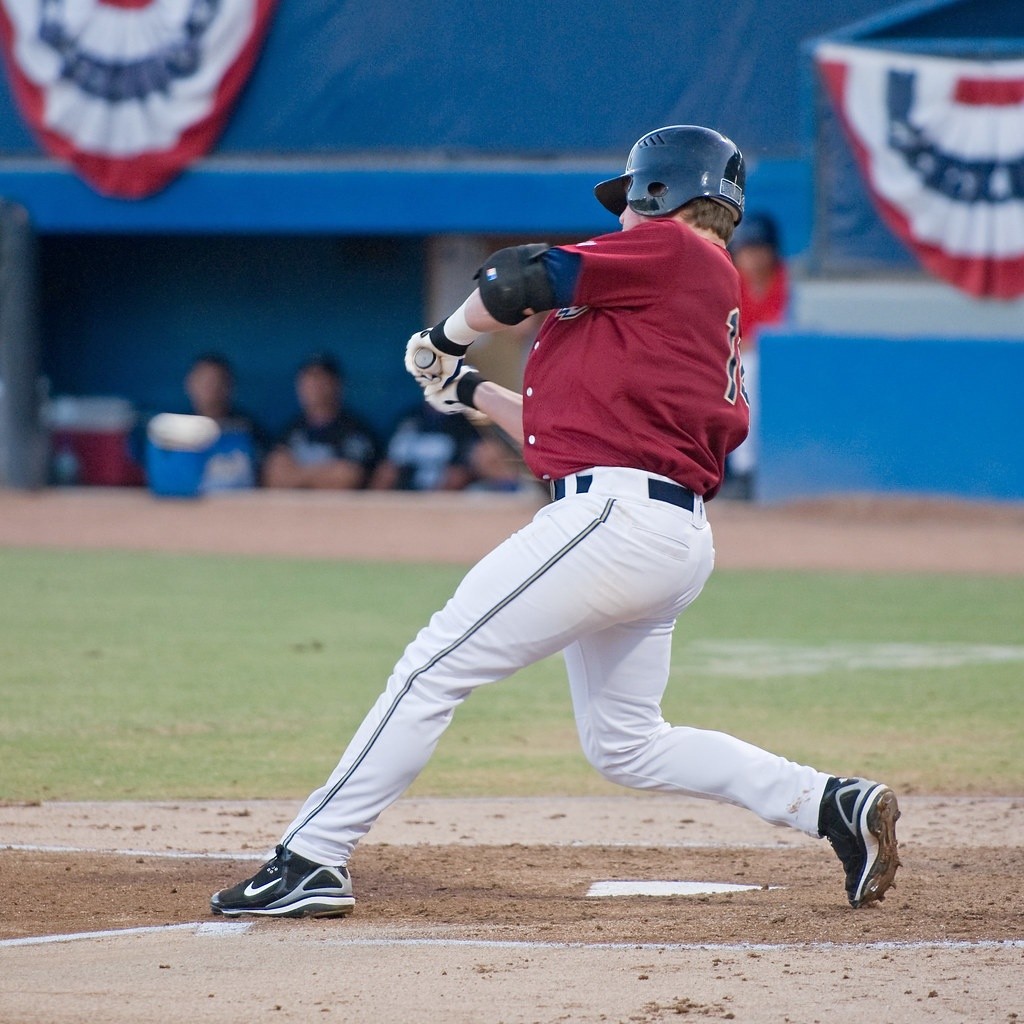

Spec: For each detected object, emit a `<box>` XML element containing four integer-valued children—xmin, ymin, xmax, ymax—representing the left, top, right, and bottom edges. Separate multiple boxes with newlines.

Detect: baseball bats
<box><xmin>412</xmin><ymin>346</ymin><xmax>492</xmax><ymax>426</ymax></box>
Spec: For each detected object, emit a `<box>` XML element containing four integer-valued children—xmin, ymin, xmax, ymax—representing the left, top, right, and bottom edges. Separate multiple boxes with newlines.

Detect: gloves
<box><xmin>405</xmin><ymin>316</ymin><xmax>474</xmax><ymax>395</ymax></box>
<box><xmin>424</xmin><ymin>366</ymin><xmax>491</xmax><ymax>415</ymax></box>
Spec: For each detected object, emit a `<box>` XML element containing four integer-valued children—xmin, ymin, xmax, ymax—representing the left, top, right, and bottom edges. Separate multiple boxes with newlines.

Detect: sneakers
<box><xmin>210</xmin><ymin>844</ymin><xmax>355</xmax><ymax>918</ymax></box>
<box><xmin>818</xmin><ymin>777</ymin><xmax>903</xmax><ymax>909</ymax></box>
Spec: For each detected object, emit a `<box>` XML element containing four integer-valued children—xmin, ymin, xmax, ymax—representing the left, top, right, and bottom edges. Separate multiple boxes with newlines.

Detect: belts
<box><xmin>550</xmin><ymin>475</ymin><xmax>702</xmax><ymax>514</ymax></box>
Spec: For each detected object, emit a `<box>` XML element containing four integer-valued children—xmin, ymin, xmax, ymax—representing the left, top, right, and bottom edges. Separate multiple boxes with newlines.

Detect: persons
<box><xmin>185</xmin><ymin>212</ymin><xmax>795</xmax><ymax>501</ymax></box>
<box><xmin>212</xmin><ymin>126</ymin><xmax>901</xmax><ymax>921</ymax></box>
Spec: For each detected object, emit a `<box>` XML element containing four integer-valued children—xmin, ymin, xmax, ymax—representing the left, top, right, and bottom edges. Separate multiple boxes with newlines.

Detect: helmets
<box><xmin>593</xmin><ymin>125</ymin><xmax>746</xmax><ymax>227</ymax></box>
